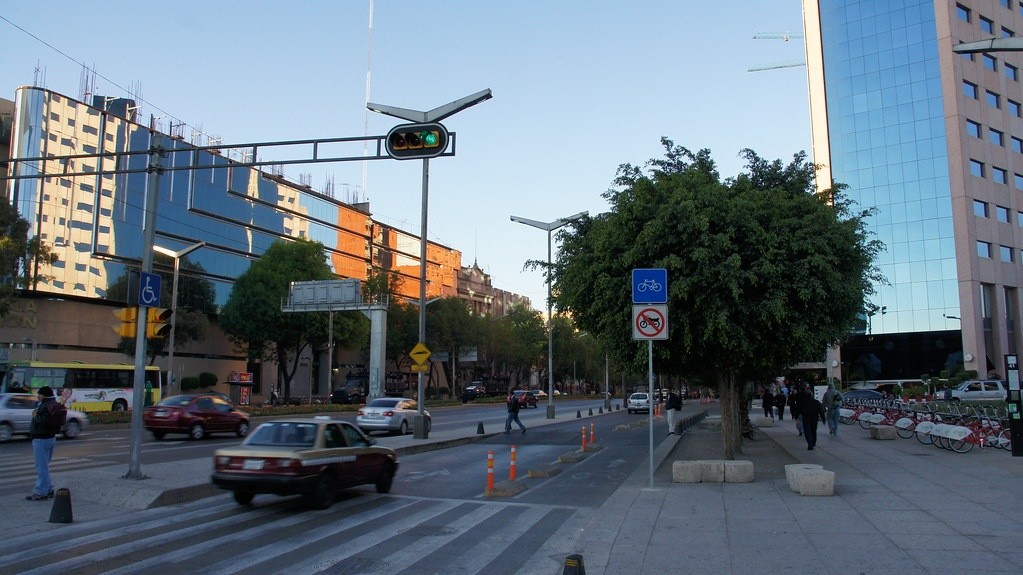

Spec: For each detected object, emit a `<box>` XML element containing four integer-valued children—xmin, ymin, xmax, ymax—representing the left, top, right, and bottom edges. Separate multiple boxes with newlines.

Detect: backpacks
<box><xmin>673</xmin><ymin>396</ymin><xmax>682</xmax><ymax>411</ymax></box>
<box><xmin>38</xmin><ymin>400</ymin><xmax>67</xmax><ymax>426</ymax></box>
<box><xmin>833</xmin><ymin>391</ymin><xmax>840</xmax><ymax>407</ymax></box>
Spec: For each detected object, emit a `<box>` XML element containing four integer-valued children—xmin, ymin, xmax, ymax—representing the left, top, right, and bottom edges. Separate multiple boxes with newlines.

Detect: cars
<box><xmin>533</xmin><ymin>389</ymin><xmax>567</xmax><ymax>397</ymax></box>
<box><xmin>211</xmin><ymin>415</ymin><xmax>400</xmax><ymax>510</ymax></box>
<box><xmin>0</xmin><ymin>392</ymin><xmax>89</xmax><ymax>443</ymax></box>
<box><xmin>356</xmin><ymin>397</ymin><xmax>432</xmax><ymax>433</ymax></box>
<box><xmin>628</xmin><ymin>392</ymin><xmax>650</xmax><ymax>413</ymax></box>
<box><xmin>142</xmin><ymin>393</ymin><xmax>250</xmax><ymax>440</ymax></box>
<box><xmin>838</xmin><ymin>389</ymin><xmax>886</xmax><ymax>400</ymax></box>
<box><xmin>506</xmin><ymin>390</ymin><xmax>538</xmax><ymax>408</ymax></box>
<box><xmin>653</xmin><ymin>389</ymin><xmax>689</xmax><ymax>397</ymax></box>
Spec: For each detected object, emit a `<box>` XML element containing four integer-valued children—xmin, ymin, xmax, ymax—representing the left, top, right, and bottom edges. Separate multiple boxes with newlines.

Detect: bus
<box><xmin>0</xmin><ymin>360</ymin><xmax>162</xmax><ymax>412</ymax></box>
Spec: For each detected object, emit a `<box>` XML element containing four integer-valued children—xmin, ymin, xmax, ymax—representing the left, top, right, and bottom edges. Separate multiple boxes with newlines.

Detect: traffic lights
<box><xmin>148</xmin><ymin>307</ymin><xmax>172</xmax><ymax>339</ymax></box>
<box><xmin>110</xmin><ymin>308</ymin><xmax>136</xmax><ymax>336</ymax></box>
<box><xmin>384</xmin><ymin>123</ymin><xmax>450</xmax><ymax>159</ymax></box>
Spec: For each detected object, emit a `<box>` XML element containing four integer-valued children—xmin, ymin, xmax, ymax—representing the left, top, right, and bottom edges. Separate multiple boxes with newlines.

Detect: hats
<box><xmin>38</xmin><ymin>386</ymin><xmax>54</xmax><ymax>396</ymax></box>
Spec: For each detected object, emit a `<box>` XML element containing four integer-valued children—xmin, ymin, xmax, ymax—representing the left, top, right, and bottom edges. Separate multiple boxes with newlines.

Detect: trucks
<box><xmin>333</xmin><ymin>369</ymin><xmax>404</xmax><ymax>403</ymax></box>
<box><xmin>462</xmin><ymin>376</ymin><xmax>508</xmax><ymax>402</ymax></box>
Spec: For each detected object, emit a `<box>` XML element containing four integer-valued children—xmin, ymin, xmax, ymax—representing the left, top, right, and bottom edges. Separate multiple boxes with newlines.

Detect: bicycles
<box><xmin>838</xmin><ymin>399</ymin><xmax>1012</xmax><ymax>454</ymax></box>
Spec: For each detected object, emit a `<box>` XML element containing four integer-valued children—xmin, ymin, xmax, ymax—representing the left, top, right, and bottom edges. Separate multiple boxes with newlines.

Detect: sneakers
<box><xmin>25</xmin><ymin>490</ymin><xmax>54</xmax><ymax>500</ymax></box>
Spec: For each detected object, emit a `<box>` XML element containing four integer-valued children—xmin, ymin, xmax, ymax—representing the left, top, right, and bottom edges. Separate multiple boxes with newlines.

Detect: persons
<box><xmin>761</xmin><ymin>375</ymin><xmax>843</xmax><ymax>436</ymax></box>
<box><xmin>664</xmin><ymin>388</ymin><xmax>678</xmax><ymax>436</ymax></box>
<box><xmin>503</xmin><ymin>392</ymin><xmax>526</xmax><ymax>434</ymax></box>
<box><xmin>800</xmin><ymin>392</ymin><xmax>826</xmax><ymax>451</ymax></box>
<box><xmin>270</xmin><ymin>383</ymin><xmax>276</xmax><ymax>405</ymax></box>
<box><xmin>943</xmin><ymin>384</ymin><xmax>953</xmax><ymax>403</ymax></box>
<box><xmin>25</xmin><ymin>386</ymin><xmax>60</xmax><ymax>501</ymax></box>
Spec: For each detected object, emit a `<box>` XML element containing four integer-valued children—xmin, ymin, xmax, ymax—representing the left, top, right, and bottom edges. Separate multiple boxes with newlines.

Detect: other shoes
<box><xmin>829</xmin><ymin>426</ymin><xmax>835</xmax><ymax>434</ymax></box>
<box><xmin>522</xmin><ymin>427</ymin><xmax>528</xmax><ymax>433</ymax></box>
<box><xmin>504</xmin><ymin>431</ymin><xmax>511</xmax><ymax>434</ymax></box>
<box><xmin>668</xmin><ymin>432</ymin><xmax>675</xmax><ymax>436</ymax></box>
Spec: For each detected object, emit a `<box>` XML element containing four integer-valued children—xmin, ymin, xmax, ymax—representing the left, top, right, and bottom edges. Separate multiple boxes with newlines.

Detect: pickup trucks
<box><xmin>933</xmin><ymin>380</ymin><xmax>1023</xmax><ymax>400</ymax></box>
